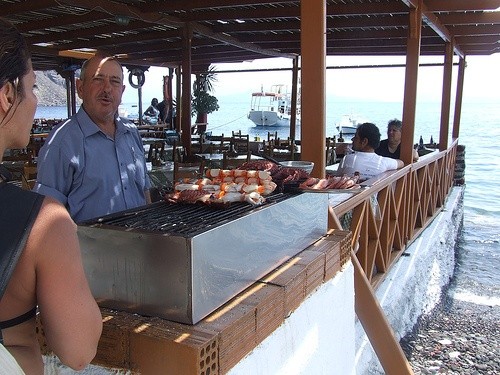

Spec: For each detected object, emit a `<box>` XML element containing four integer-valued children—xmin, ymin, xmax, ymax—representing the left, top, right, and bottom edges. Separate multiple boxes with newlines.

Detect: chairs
<box><xmin>0</xmin><ymin>119</ymin><xmax>436</xmax><ymax>192</ymax></box>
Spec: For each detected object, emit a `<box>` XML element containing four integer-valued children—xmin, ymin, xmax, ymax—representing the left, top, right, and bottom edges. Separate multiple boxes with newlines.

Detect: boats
<box><xmin>246</xmin><ymin>79</ymin><xmax>302</xmax><ymax>127</ymax></box>
<box><xmin>335</xmin><ymin>110</ymin><xmax>363</xmax><ymax>134</ymax></box>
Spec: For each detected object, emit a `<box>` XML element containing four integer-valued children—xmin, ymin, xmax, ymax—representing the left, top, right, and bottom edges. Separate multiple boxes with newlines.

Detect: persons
<box><xmin>0</xmin><ymin>20</ymin><xmax>102</xmax><ymax>375</ymax></box>
<box><xmin>144</xmin><ymin>98</ymin><xmax>160</xmax><ymax>131</ymax></box>
<box><xmin>32</xmin><ymin>56</ymin><xmax>152</xmax><ymax>224</ymax></box>
<box><xmin>336</xmin><ymin>118</ymin><xmax>419</xmax><ymax>222</ymax></box>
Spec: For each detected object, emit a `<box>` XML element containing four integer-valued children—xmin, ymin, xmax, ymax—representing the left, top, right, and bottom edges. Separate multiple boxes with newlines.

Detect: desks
<box><xmin>259</xmin><ymin>145</ymin><xmax>336</xmax><ymax>161</ymax></box>
<box><xmin>195</xmin><ymin>153</ymin><xmax>266</xmax><ymax>165</ymax></box>
<box><xmin>29</xmin><ymin>133</ymin><xmax>49</xmax><ymax>157</ymax></box>
<box><xmin>137</xmin><ymin>124</ymin><xmax>168</xmax><ymax>132</ymax></box>
